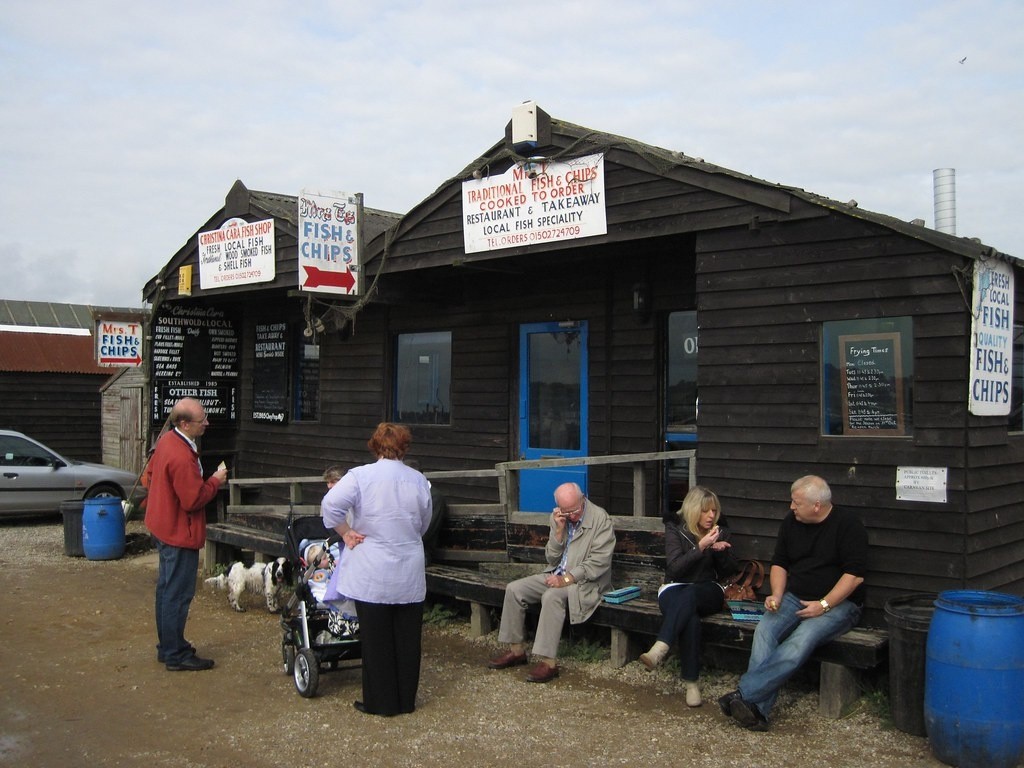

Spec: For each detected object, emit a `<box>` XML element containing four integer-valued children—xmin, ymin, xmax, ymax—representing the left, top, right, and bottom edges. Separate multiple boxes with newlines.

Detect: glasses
<box><xmin>188</xmin><ymin>412</ymin><xmax>208</xmax><ymax>423</ymax></box>
<box><xmin>559</xmin><ymin>506</ymin><xmax>580</xmax><ymax>516</ymax></box>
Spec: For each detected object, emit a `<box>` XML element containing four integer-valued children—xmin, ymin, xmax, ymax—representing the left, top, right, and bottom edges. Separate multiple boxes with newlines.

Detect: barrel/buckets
<box><xmin>924</xmin><ymin>589</ymin><xmax>1024</xmax><ymax>768</ymax></box>
<box><xmin>82</xmin><ymin>497</ymin><xmax>125</xmax><ymax>560</ymax></box>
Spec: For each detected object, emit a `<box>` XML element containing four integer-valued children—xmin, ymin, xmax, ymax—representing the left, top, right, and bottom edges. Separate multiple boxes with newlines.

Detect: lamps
<box><xmin>622</xmin><ymin>265</ymin><xmax>654</xmax><ymax>324</ymax></box>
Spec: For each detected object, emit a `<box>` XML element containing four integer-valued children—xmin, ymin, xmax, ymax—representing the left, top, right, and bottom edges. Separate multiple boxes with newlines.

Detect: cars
<box><xmin>0</xmin><ymin>429</ymin><xmax>148</xmax><ymax>517</ymax></box>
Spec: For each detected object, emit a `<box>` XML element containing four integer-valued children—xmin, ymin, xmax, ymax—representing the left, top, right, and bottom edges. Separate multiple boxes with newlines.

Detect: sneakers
<box><xmin>167</xmin><ymin>656</ymin><xmax>214</xmax><ymax>671</ymax></box>
<box><xmin>157</xmin><ymin>647</ymin><xmax>196</xmax><ymax>660</ymax></box>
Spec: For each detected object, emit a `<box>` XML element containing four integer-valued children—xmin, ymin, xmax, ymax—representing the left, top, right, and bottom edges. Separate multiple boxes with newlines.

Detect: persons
<box><xmin>141</xmin><ymin>397</ymin><xmax>228</xmax><ymax>670</ymax></box>
<box><xmin>401</xmin><ymin>458</ymin><xmax>448</xmax><ymax>567</ymax></box>
<box><xmin>324</xmin><ymin>464</ymin><xmax>355</xmax><ymax>530</ymax></box>
<box><xmin>303</xmin><ymin>542</ymin><xmax>359</xmax><ymax>639</ymax></box>
<box><xmin>488</xmin><ymin>483</ymin><xmax>617</xmax><ymax>683</ymax></box>
<box><xmin>318</xmin><ymin>422</ymin><xmax>433</xmax><ymax>719</ymax></box>
<box><xmin>718</xmin><ymin>475</ymin><xmax>869</xmax><ymax>731</ymax></box>
<box><xmin>638</xmin><ymin>486</ymin><xmax>735</xmax><ymax>708</ymax></box>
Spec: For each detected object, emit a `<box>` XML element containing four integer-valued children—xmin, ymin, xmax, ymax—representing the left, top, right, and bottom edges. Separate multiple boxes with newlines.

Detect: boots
<box><xmin>685</xmin><ymin>679</ymin><xmax>702</xmax><ymax>707</ymax></box>
<box><xmin>640</xmin><ymin>641</ymin><xmax>670</xmax><ymax>672</ymax></box>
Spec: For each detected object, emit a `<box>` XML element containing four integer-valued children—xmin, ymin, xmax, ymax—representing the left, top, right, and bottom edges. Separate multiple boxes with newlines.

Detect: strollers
<box><xmin>281</xmin><ymin>514</ymin><xmax>361</xmax><ymax>697</ymax></box>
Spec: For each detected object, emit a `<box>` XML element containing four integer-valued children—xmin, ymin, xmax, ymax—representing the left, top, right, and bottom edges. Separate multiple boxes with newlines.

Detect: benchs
<box><xmin>421</xmin><ymin>565</ymin><xmax>887</xmax><ymax>723</ymax></box>
<box><xmin>204</xmin><ymin>522</ymin><xmax>290</xmax><ymax>572</ymax></box>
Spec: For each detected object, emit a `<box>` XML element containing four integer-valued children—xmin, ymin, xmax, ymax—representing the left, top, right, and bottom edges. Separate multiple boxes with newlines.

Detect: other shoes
<box><xmin>354</xmin><ymin>700</ymin><xmax>367</xmax><ymax>712</ymax></box>
<box><xmin>730</xmin><ymin>698</ymin><xmax>769</xmax><ymax>731</ymax></box>
<box><xmin>718</xmin><ymin>690</ymin><xmax>742</xmax><ymax>716</ymax></box>
<box><xmin>489</xmin><ymin>651</ymin><xmax>527</xmax><ymax>669</ymax></box>
<box><xmin>526</xmin><ymin>662</ymin><xmax>559</xmax><ymax>682</ymax></box>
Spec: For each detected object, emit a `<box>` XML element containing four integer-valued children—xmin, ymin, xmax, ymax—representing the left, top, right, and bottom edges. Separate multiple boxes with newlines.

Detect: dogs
<box><xmin>205</xmin><ymin>556</ymin><xmax>291</xmax><ymax>613</ymax></box>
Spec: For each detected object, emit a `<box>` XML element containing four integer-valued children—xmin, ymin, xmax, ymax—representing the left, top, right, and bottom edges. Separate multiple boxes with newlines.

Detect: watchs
<box><xmin>819</xmin><ymin>599</ymin><xmax>831</xmax><ymax>613</ymax></box>
<box><xmin>562</xmin><ymin>574</ymin><xmax>570</xmax><ymax>585</ymax></box>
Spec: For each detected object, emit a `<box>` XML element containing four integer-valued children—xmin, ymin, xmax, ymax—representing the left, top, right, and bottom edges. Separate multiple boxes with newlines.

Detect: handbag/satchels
<box><xmin>723</xmin><ymin>560</ymin><xmax>765</xmax><ymax>608</ymax></box>
